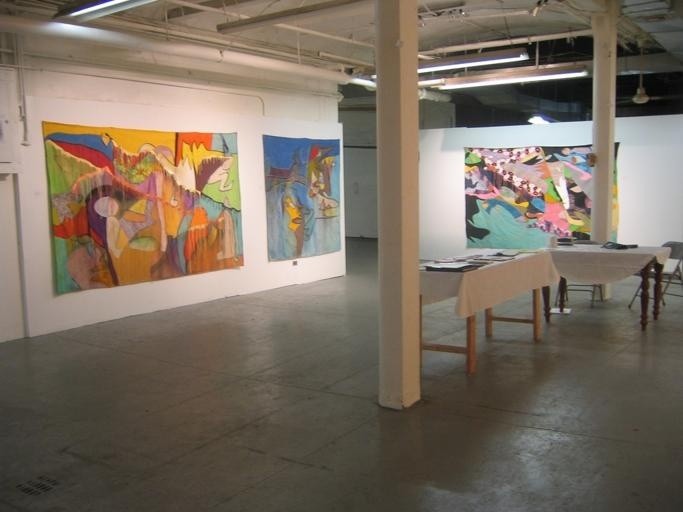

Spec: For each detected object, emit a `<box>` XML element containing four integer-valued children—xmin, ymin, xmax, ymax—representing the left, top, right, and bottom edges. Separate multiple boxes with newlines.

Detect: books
<box><xmin>423</xmin><ymin>248</ymin><xmax>543</xmax><ymax>273</ymax></box>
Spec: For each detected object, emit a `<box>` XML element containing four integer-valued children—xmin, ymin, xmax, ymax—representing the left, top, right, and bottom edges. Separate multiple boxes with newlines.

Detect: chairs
<box><xmin>627</xmin><ymin>240</ymin><xmax>683</xmax><ymax>309</ymax></box>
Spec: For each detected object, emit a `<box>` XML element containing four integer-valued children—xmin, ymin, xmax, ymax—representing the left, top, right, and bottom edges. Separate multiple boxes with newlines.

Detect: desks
<box><xmin>420</xmin><ymin>243</ymin><xmax>673</xmax><ymax>377</ymax></box>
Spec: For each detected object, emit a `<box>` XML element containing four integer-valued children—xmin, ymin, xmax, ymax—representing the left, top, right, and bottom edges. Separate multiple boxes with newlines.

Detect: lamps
<box><xmin>632</xmin><ymin>39</ymin><xmax>649</xmax><ymax>104</ymax></box>
